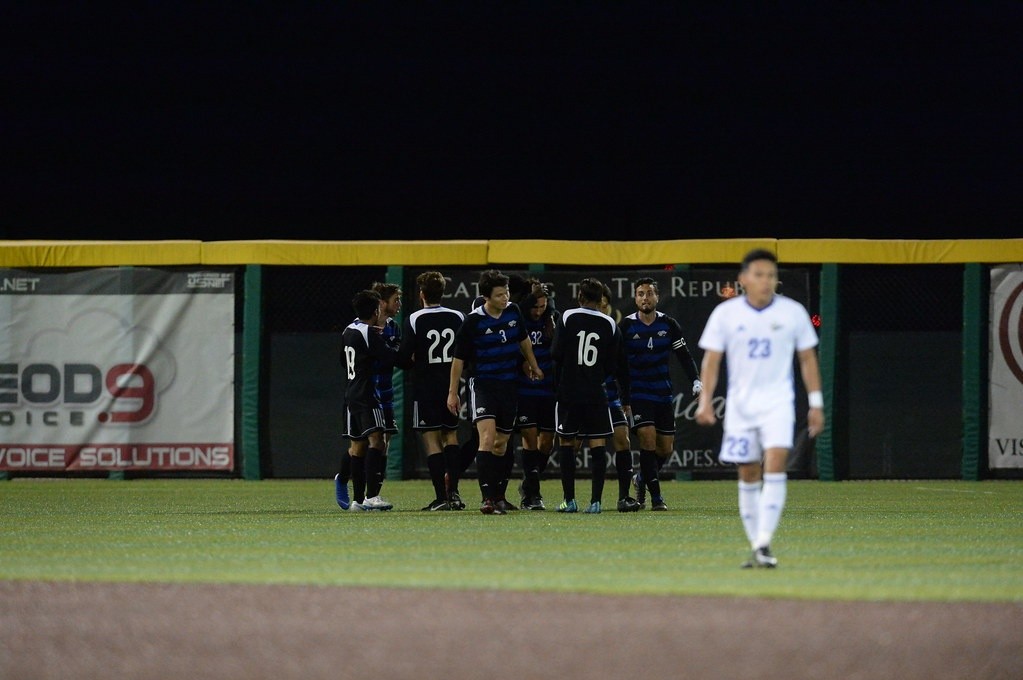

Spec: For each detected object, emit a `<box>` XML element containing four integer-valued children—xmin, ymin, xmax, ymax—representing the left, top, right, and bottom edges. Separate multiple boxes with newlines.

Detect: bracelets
<box><xmin>807</xmin><ymin>390</ymin><xmax>825</xmax><ymax>408</ymax></box>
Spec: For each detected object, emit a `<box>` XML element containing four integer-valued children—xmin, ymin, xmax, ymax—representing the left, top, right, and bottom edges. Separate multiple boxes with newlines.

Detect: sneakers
<box><xmin>555</xmin><ymin>499</ymin><xmax>577</xmax><ymax>513</ymax></box>
<box><xmin>363</xmin><ymin>496</ymin><xmax>393</xmax><ymax>512</ymax></box>
<box><xmin>583</xmin><ymin>501</ymin><xmax>602</xmax><ymax>513</ymax></box>
<box><xmin>521</xmin><ymin>496</ymin><xmax>545</xmax><ymax>510</ymax></box>
<box><xmin>448</xmin><ymin>493</ymin><xmax>465</xmax><ymax>510</ymax></box>
<box><xmin>335</xmin><ymin>473</ymin><xmax>350</xmax><ymax>509</ymax></box>
<box><xmin>351</xmin><ymin>501</ymin><xmax>367</xmax><ymax>512</ymax></box>
<box><xmin>421</xmin><ymin>499</ymin><xmax>450</xmax><ymax>513</ymax></box>
<box><xmin>480</xmin><ymin>498</ymin><xmax>495</xmax><ymax>514</ymax></box>
<box><xmin>493</xmin><ymin>498</ymin><xmax>520</xmax><ymax>511</ymax></box>
<box><xmin>740</xmin><ymin>553</ymin><xmax>761</xmax><ymax>568</ymax></box>
<box><xmin>651</xmin><ymin>501</ymin><xmax>668</xmax><ymax>511</ymax></box>
<box><xmin>617</xmin><ymin>497</ymin><xmax>638</xmax><ymax>513</ymax></box>
<box><xmin>755</xmin><ymin>550</ymin><xmax>778</xmax><ymax>567</ymax></box>
<box><xmin>634</xmin><ymin>501</ymin><xmax>647</xmax><ymax>511</ymax></box>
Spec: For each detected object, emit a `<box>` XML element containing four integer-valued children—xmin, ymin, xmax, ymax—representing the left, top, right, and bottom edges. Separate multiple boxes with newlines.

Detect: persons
<box><xmin>618</xmin><ymin>278</ymin><xmax>701</xmax><ymax>512</ymax></box>
<box><xmin>335</xmin><ymin>284</ymin><xmax>403</xmax><ymax>511</ymax></box>
<box><xmin>395</xmin><ymin>271</ymin><xmax>466</xmax><ymax>511</ymax></box>
<box><xmin>447</xmin><ymin>271</ymin><xmax>545</xmax><ymax>515</ymax></box>
<box><xmin>694</xmin><ymin>248</ymin><xmax>824</xmax><ymax>570</ymax></box>
<box><xmin>552</xmin><ymin>279</ymin><xmax>642</xmax><ymax>513</ymax></box>
<box><xmin>446</xmin><ymin>272</ymin><xmax>560</xmax><ymax>510</ymax></box>
